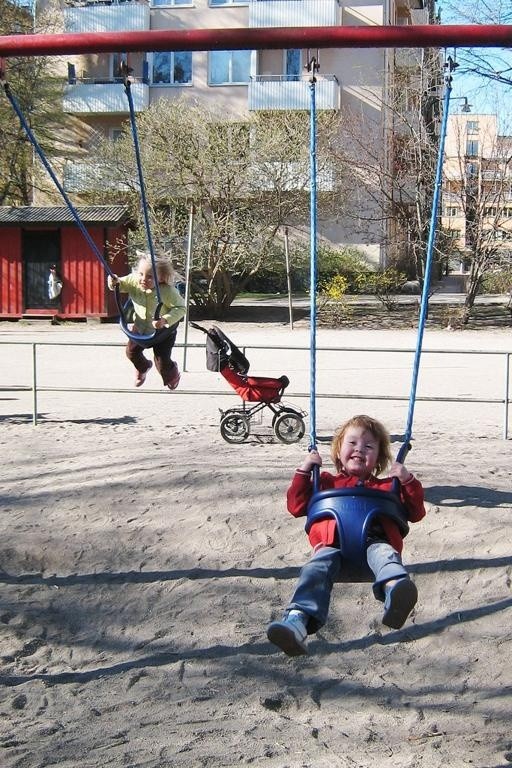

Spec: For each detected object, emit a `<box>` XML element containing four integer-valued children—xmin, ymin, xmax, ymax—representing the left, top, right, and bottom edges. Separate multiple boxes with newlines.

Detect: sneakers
<box><xmin>167</xmin><ymin>362</ymin><xmax>180</xmax><ymax>389</ymax></box>
<box><xmin>267</xmin><ymin>618</ymin><xmax>311</xmax><ymax>657</ymax></box>
<box><xmin>136</xmin><ymin>361</ymin><xmax>152</xmax><ymax>387</ymax></box>
<box><xmin>381</xmin><ymin>578</ymin><xmax>418</xmax><ymax>630</ymax></box>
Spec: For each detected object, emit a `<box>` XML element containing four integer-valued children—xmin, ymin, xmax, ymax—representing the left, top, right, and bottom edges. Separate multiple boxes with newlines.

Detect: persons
<box><xmin>106</xmin><ymin>243</ymin><xmax>189</xmax><ymax>390</ymax></box>
<box><xmin>268</xmin><ymin>414</ymin><xmax>429</xmax><ymax>658</ymax></box>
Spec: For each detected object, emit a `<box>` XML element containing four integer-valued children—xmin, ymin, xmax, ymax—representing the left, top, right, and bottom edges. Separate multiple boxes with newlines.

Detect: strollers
<box><xmin>188</xmin><ymin>321</ymin><xmax>308</xmax><ymax>443</ymax></box>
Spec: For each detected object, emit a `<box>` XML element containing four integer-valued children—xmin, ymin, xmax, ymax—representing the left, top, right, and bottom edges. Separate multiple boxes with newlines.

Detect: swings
<box><xmin>302</xmin><ymin>44</ymin><xmax>459</xmax><ymax>583</ymax></box>
<box><xmin>0</xmin><ymin>56</ymin><xmax>167</xmax><ymax>346</ymax></box>
